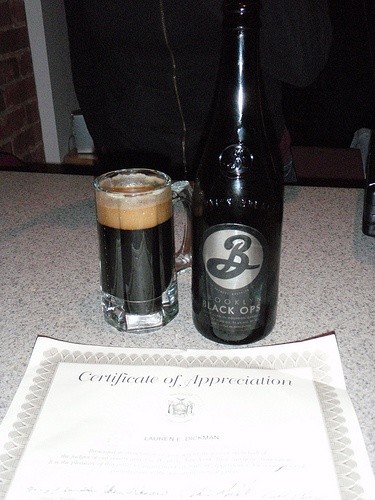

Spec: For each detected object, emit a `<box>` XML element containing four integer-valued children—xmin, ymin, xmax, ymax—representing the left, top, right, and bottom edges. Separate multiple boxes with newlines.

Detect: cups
<box><xmin>92</xmin><ymin>168</ymin><xmax>192</xmax><ymax>333</ymax></box>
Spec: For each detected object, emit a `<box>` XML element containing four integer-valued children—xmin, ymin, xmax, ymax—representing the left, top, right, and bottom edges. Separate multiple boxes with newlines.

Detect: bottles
<box><xmin>188</xmin><ymin>3</ymin><xmax>286</xmax><ymax>346</ymax></box>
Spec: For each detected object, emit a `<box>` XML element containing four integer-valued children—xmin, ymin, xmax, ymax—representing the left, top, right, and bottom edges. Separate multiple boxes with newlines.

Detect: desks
<box><xmin>0</xmin><ymin>169</ymin><xmax>375</xmax><ymax>476</ymax></box>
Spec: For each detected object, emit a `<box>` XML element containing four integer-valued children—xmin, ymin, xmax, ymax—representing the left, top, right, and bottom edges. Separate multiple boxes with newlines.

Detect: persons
<box><xmin>64</xmin><ymin>0</ymin><xmax>332</xmax><ymax>185</ymax></box>
